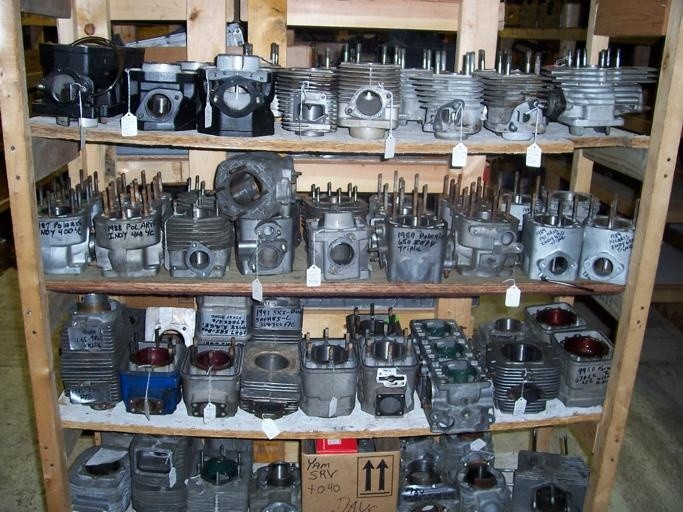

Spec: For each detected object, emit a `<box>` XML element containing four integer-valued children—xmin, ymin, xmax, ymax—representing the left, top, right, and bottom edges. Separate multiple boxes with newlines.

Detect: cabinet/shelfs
<box><xmin>0</xmin><ymin>0</ymin><xmax>683</xmax><ymax>512</ymax></box>
